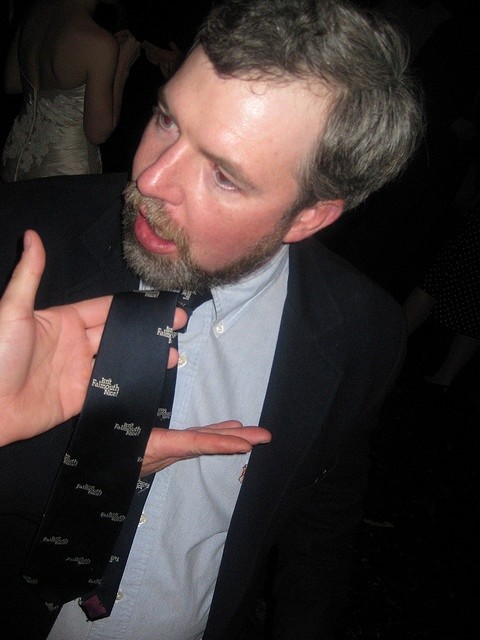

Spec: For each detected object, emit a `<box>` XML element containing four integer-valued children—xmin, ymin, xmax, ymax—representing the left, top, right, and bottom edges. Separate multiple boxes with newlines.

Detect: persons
<box><xmin>3</xmin><ymin>16</ymin><xmax>142</xmax><ymax>183</ymax></box>
<box><xmin>405</xmin><ymin>205</ymin><xmax>479</xmax><ymax>386</ymax></box>
<box><xmin>0</xmin><ymin>227</ymin><xmax>190</xmax><ymax>449</ymax></box>
<box><xmin>0</xmin><ymin>1</ymin><xmax>427</xmax><ymax>640</ymax></box>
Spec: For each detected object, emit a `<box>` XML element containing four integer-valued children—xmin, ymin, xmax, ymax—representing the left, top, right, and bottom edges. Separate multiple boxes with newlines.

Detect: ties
<box><xmin>24</xmin><ymin>271</ymin><xmax>214</xmax><ymax>622</ymax></box>
